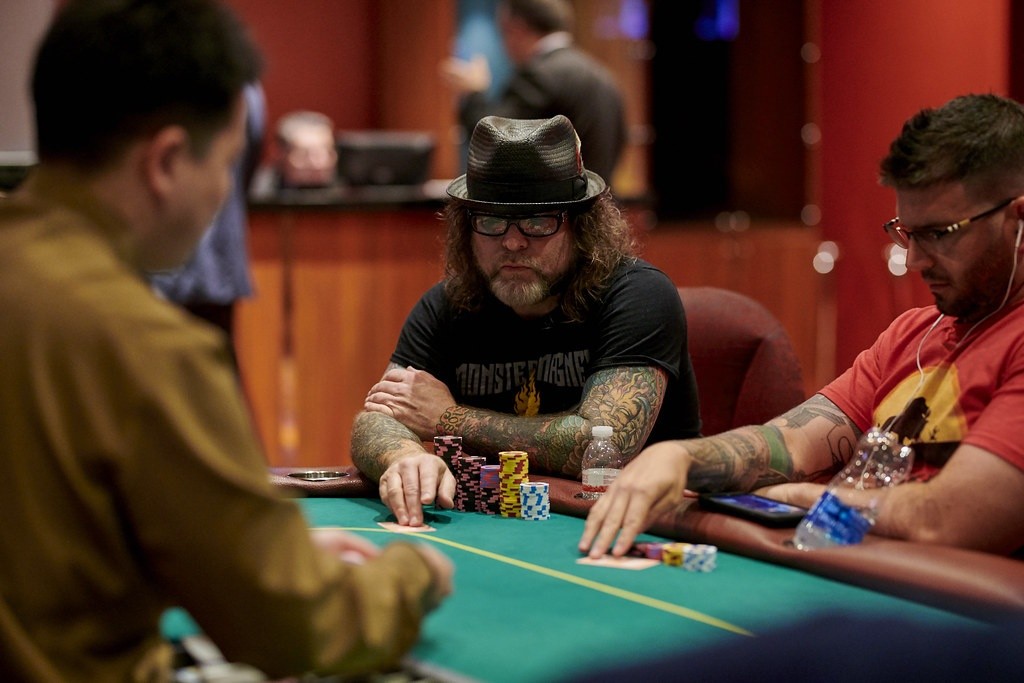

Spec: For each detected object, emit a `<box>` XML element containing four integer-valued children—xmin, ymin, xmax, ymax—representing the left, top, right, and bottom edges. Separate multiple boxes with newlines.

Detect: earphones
<box><xmin>1015</xmin><ymin>219</ymin><xmax>1024</xmax><ymax>249</ymax></box>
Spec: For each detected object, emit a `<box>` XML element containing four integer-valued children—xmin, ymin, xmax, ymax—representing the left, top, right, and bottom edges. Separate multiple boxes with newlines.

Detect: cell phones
<box><xmin>697</xmin><ymin>493</ymin><xmax>811</xmax><ymax>529</ymax></box>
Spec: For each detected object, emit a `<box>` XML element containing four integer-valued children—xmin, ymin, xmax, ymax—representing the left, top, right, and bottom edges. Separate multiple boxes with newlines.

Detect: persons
<box><xmin>350</xmin><ymin>115</ymin><xmax>701</xmax><ymax>527</ymax></box>
<box><xmin>144</xmin><ymin>81</ymin><xmax>265</xmax><ymax>381</ymax></box>
<box><xmin>578</xmin><ymin>93</ymin><xmax>1024</xmax><ymax>558</ymax></box>
<box><xmin>0</xmin><ymin>0</ymin><xmax>453</xmax><ymax>683</ymax></box>
<box><xmin>273</xmin><ymin>111</ymin><xmax>338</xmax><ymax>189</ymax></box>
<box><xmin>439</xmin><ymin>0</ymin><xmax>628</xmax><ymax>186</ymax></box>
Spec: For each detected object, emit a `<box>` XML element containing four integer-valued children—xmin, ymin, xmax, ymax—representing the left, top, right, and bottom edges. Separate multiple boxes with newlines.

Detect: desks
<box><xmin>44</xmin><ymin>464</ymin><xmax>1024</xmax><ymax>683</ymax></box>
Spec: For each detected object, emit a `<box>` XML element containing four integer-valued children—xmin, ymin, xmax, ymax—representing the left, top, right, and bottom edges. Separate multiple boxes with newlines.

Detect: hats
<box><xmin>446</xmin><ymin>115</ymin><xmax>606</xmax><ymax>216</ymax></box>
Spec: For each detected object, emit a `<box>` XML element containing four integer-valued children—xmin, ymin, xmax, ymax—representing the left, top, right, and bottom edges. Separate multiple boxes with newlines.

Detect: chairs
<box><xmin>659</xmin><ymin>282</ymin><xmax>810</xmax><ymax>469</ymax></box>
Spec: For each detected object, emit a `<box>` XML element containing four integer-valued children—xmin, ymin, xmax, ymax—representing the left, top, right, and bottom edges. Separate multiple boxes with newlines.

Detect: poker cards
<box><xmin>377</xmin><ymin>521</ymin><xmax>437</xmax><ymax>533</ymax></box>
<box><xmin>576</xmin><ymin>552</ymin><xmax>661</xmax><ymax>572</ymax></box>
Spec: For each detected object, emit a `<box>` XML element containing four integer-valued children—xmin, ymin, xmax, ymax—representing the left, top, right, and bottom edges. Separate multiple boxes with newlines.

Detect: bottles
<box><xmin>582</xmin><ymin>426</ymin><xmax>624</xmax><ymax>501</ymax></box>
<box><xmin>793</xmin><ymin>427</ymin><xmax>916</xmax><ymax>551</ymax></box>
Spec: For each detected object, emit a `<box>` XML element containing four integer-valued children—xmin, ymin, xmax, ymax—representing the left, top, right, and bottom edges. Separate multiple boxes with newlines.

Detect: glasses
<box><xmin>882</xmin><ymin>196</ymin><xmax>1018</xmax><ymax>254</ymax></box>
<box><xmin>464</xmin><ymin>205</ymin><xmax>578</xmax><ymax>238</ymax></box>
